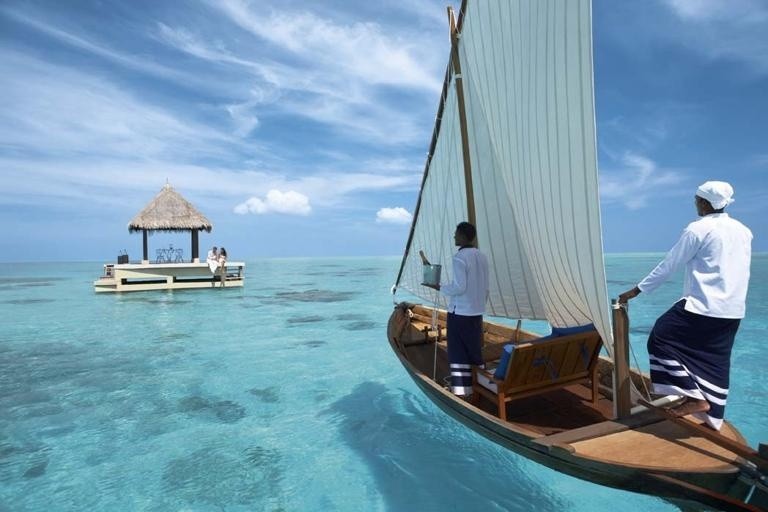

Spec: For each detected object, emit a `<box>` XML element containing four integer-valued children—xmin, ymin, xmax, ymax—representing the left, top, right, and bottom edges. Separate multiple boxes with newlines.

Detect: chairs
<box><xmin>156</xmin><ymin>249</ymin><xmax>184</xmax><ymax>264</ymax></box>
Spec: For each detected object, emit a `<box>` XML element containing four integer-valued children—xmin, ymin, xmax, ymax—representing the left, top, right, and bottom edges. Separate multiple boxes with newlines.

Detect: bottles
<box><xmin>419</xmin><ymin>251</ymin><xmax>431</xmax><ymax>266</ymax></box>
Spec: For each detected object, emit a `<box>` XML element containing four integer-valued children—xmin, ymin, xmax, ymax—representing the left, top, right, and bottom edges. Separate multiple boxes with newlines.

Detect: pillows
<box><xmin>488</xmin><ymin>323</ymin><xmax>604</xmax><ymax>385</ymax></box>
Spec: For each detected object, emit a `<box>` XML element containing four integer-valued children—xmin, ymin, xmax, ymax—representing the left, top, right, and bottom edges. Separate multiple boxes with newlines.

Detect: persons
<box><xmin>420</xmin><ymin>222</ymin><xmax>490</xmax><ymax>402</ymax></box>
<box><xmin>206</xmin><ymin>245</ymin><xmax>220</xmax><ymax>274</ymax></box>
<box><xmin>618</xmin><ymin>180</ymin><xmax>754</xmax><ymax>432</ymax></box>
<box><xmin>218</xmin><ymin>246</ymin><xmax>228</xmax><ymax>270</ymax></box>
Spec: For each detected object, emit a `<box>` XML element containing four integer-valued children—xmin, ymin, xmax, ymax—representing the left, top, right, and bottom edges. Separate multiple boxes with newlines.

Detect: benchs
<box><xmin>472</xmin><ymin>332</ymin><xmax>604</xmax><ymax>422</ymax></box>
